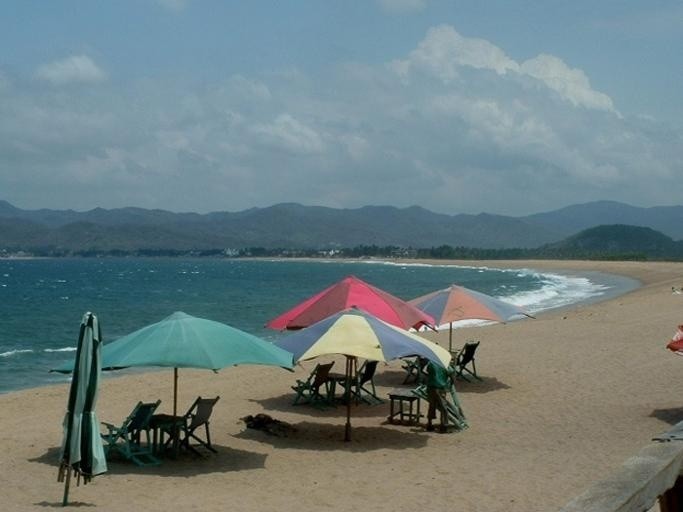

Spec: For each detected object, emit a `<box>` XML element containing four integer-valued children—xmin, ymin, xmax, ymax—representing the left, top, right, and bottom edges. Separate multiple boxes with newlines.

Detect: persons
<box><xmin>425</xmin><ymin>358</ymin><xmax>450</xmax><ymax>433</ymax></box>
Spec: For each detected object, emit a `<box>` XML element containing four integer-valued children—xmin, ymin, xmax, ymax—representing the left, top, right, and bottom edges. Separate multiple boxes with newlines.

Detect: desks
<box><xmin>144</xmin><ymin>411</ymin><xmax>182</xmax><ymax>459</ymax></box>
<box><xmin>384</xmin><ymin>386</ymin><xmax>421</xmax><ymax>424</ymax></box>
<box><xmin>322</xmin><ymin>372</ymin><xmax>349</xmax><ymax>405</ymax></box>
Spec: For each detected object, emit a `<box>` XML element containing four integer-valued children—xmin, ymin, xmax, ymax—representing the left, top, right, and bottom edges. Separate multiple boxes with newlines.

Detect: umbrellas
<box><xmin>55</xmin><ymin>308</ymin><xmax>107</xmax><ymax>508</ymax></box>
<box><xmin>403</xmin><ymin>282</ymin><xmax>533</xmax><ymax>357</ymax></box>
<box><xmin>262</xmin><ymin>273</ymin><xmax>437</xmax><ymax>400</ymax></box>
<box><xmin>268</xmin><ymin>304</ymin><xmax>451</xmax><ymax>444</ymax></box>
<box><xmin>47</xmin><ymin>310</ymin><xmax>296</xmax><ymax>454</ymax></box>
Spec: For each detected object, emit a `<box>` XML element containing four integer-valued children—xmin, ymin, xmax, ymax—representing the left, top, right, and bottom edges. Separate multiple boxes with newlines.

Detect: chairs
<box><xmin>291</xmin><ymin>361</ymin><xmax>339</xmax><ymax>411</ymax></box>
<box><xmin>146</xmin><ymin>396</ymin><xmax>221</xmax><ymax>462</ymax></box>
<box><xmin>336</xmin><ymin>359</ymin><xmax>385</xmax><ymax>407</ymax></box>
<box><xmin>398</xmin><ymin>356</ymin><xmax>428</xmax><ymax>384</ymax></box>
<box><xmin>453</xmin><ymin>339</ymin><xmax>484</xmax><ymax>386</ymax></box>
<box><xmin>409</xmin><ymin>383</ymin><xmax>470</xmax><ymax>431</ymax></box>
<box><xmin>99</xmin><ymin>397</ymin><xmax>163</xmax><ymax>468</ymax></box>
